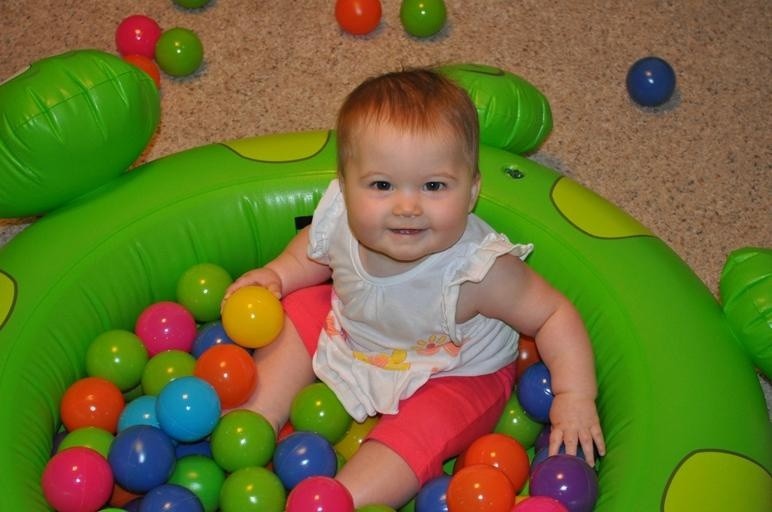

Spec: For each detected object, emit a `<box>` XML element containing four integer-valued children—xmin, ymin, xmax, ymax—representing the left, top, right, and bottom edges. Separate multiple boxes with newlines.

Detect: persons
<box><xmin>218</xmin><ymin>66</ymin><xmax>608</xmax><ymax>510</ymax></box>
